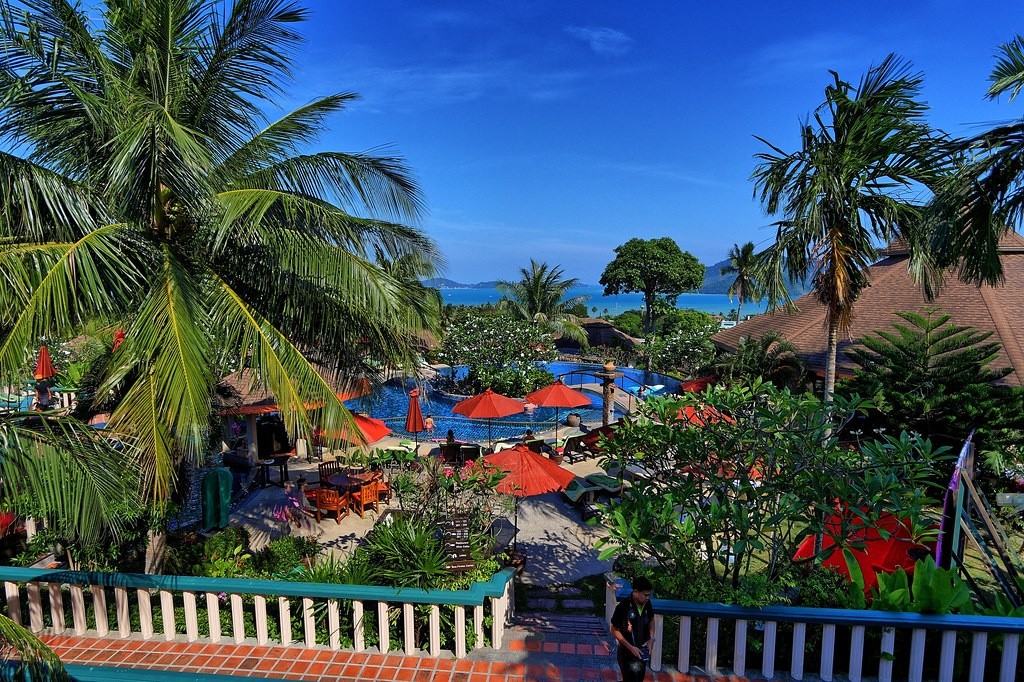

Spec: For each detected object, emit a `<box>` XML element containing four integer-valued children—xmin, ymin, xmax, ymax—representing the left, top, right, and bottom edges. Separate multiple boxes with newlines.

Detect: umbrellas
<box><xmin>304</xmin><ymin>410</ymin><xmax>393</xmax><ymax>481</ymax></box>
<box><xmin>473</xmin><ymin>443</ymin><xmax>576</xmax><ymax>551</ymax></box>
<box><xmin>405</xmin><ymin>388</ymin><xmax>425</xmax><ymax>461</ymax></box>
<box><xmin>452</xmin><ymin>387</ymin><xmax>525</xmax><ymax>455</ymax></box>
<box><xmin>524</xmin><ymin>378</ymin><xmax>591</xmax><ymax>450</ymax></box>
<box><xmin>34</xmin><ymin>337</ymin><xmax>57</xmax><ymax>382</ymax></box>
<box><xmin>667</xmin><ymin>375</ymin><xmax>771</xmax><ymax>482</ymax></box>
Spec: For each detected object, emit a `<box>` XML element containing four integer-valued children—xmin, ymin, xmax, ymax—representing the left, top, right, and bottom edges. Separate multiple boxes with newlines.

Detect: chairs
<box><xmin>544</xmin><ymin>433</ymin><xmax>587</xmax><ymax>465</ymax></box>
<box><xmin>439</xmin><ymin>441</ymin><xmax>460</xmax><ymax>469</ymax></box>
<box><xmin>584</xmin><ymin>472</ymin><xmax>627</xmax><ymax>508</ymax></box>
<box><xmin>624</xmin><ymin>446</ymin><xmax>691</xmax><ymax>493</ymax></box>
<box><xmin>300</xmin><ymin>461</ymin><xmax>392</xmax><ymax>525</ymax></box>
<box><xmin>558</xmin><ymin>477</ymin><xmax>603</xmax><ymax>521</ymax></box>
<box><xmin>385</xmin><ymin>440</ymin><xmax>420</xmax><ymax>460</ymax></box>
<box><xmin>460</xmin><ymin>444</ymin><xmax>483</xmax><ymax>467</ymax></box>
<box><xmin>524</xmin><ymin>437</ymin><xmax>544</xmax><ymax>456</ymax></box>
<box><xmin>0</xmin><ymin>381</ymin><xmax>35</xmax><ymax>406</ymax></box>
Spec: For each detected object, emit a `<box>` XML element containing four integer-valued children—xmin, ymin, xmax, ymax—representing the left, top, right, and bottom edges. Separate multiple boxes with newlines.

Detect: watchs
<box><xmin>650</xmin><ymin>637</ymin><xmax>656</xmax><ymax>642</ymax></box>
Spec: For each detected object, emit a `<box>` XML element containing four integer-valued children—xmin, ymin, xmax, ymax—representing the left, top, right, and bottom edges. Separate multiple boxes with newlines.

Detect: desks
<box><xmin>388</xmin><ymin>447</ymin><xmax>408</xmax><ymax>452</ymax></box>
<box><xmin>259</xmin><ymin>452</ymin><xmax>293</xmax><ymax>488</ymax></box>
<box><xmin>328</xmin><ymin>468</ymin><xmax>374</xmax><ymax>507</ymax></box>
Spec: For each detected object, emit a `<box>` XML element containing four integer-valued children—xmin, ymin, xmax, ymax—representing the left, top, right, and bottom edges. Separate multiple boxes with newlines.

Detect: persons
<box><xmin>424</xmin><ymin>413</ymin><xmax>436</xmax><ymax>432</ymax></box>
<box><xmin>446</xmin><ymin>430</ymin><xmax>456</xmax><ymax>444</ymax></box>
<box><xmin>611</xmin><ymin>577</ymin><xmax>656</xmax><ymax>682</ymax></box>
<box><xmin>523</xmin><ymin>429</ymin><xmax>535</xmax><ymax>442</ymax></box>
<box><xmin>637</xmin><ymin>384</ymin><xmax>647</xmax><ymax>396</ymax></box>
